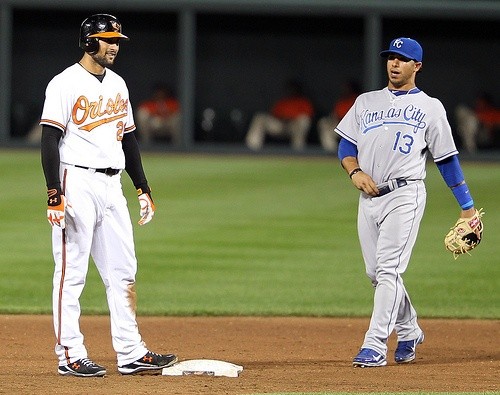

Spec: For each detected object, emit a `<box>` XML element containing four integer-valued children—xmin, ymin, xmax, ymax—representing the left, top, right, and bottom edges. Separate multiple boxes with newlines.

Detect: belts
<box><xmin>75</xmin><ymin>165</ymin><xmax>119</xmax><ymax>177</ymax></box>
<box><xmin>370</xmin><ymin>179</ymin><xmax>407</xmax><ymax>198</ymax></box>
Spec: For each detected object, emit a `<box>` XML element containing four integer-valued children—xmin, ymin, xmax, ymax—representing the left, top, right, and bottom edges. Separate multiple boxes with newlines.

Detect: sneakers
<box><xmin>117</xmin><ymin>350</ymin><xmax>178</xmax><ymax>376</ymax></box>
<box><xmin>58</xmin><ymin>357</ymin><xmax>106</xmax><ymax>377</ymax></box>
<box><xmin>352</xmin><ymin>348</ymin><xmax>387</xmax><ymax>368</ymax></box>
<box><xmin>393</xmin><ymin>333</ymin><xmax>424</xmax><ymax>363</ymax></box>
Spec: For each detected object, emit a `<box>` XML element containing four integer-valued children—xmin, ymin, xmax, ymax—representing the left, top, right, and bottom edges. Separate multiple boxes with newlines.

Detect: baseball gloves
<box><xmin>443</xmin><ymin>207</ymin><xmax>487</xmax><ymax>257</ymax></box>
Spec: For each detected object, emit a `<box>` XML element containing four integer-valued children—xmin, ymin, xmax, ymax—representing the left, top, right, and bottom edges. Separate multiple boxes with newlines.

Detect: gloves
<box><xmin>137</xmin><ymin>188</ymin><xmax>155</xmax><ymax>225</ymax></box>
<box><xmin>47</xmin><ymin>186</ymin><xmax>75</xmax><ymax>229</ymax></box>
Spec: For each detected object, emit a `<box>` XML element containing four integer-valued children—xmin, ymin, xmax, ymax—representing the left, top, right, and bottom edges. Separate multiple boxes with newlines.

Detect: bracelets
<box><xmin>349</xmin><ymin>168</ymin><xmax>362</xmax><ymax>179</ymax></box>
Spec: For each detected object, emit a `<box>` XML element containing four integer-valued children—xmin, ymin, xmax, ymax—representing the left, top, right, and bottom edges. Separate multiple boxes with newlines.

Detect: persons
<box><xmin>136</xmin><ymin>76</ymin><xmax>500</xmax><ymax>151</ymax></box>
<box><xmin>43</xmin><ymin>14</ymin><xmax>177</xmax><ymax>376</ymax></box>
<box><xmin>334</xmin><ymin>37</ymin><xmax>484</xmax><ymax>368</ymax></box>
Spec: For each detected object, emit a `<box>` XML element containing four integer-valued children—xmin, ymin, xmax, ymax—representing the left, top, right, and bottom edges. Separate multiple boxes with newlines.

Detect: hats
<box><xmin>379</xmin><ymin>37</ymin><xmax>423</xmax><ymax>62</ymax></box>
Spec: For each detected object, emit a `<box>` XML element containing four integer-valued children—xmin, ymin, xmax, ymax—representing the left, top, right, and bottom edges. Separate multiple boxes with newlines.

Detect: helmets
<box><xmin>79</xmin><ymin>14</ymin><xmax>128</xmax><ymax>55</ymax></box>
